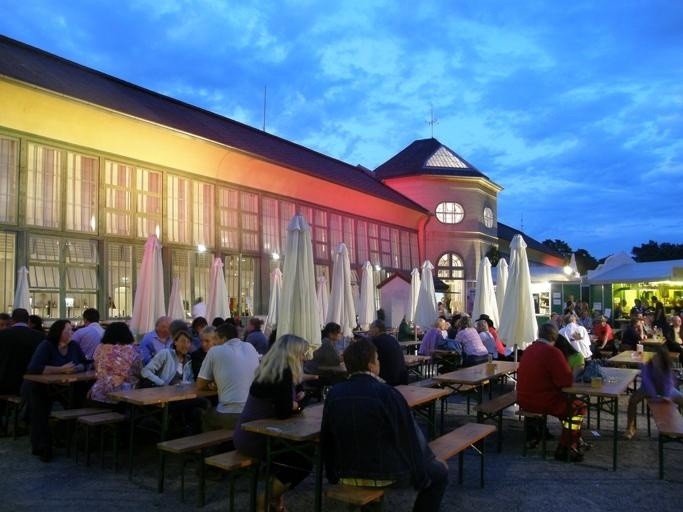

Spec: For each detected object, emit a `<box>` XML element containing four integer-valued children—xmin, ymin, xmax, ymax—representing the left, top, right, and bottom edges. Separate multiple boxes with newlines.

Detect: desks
<box><xmin>23</xmin><ymin>317</ymin><xmax>666</xmax><ymax>441</ymax></box>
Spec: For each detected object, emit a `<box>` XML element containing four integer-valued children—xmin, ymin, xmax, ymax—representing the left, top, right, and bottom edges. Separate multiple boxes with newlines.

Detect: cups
<box><xmin>322</xmin><ymin>385</ymin><xmax>332</xmax><ymax>400</ymax></box>
<box><xmin>176</xmin><ymin>380</ymin><xmax>191</xmax><ymax>392</ymax></box>
<box><xmin>590</xmin><ymin>376</ymin><xmax>602</xmax><ymax>388</ymax></box>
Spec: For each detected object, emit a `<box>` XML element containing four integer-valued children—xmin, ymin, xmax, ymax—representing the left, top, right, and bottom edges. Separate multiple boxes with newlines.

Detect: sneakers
<box><xmin>555</xmin><ymin>434</ymin><xmax>592</xmax><ymax>463</ymax></box>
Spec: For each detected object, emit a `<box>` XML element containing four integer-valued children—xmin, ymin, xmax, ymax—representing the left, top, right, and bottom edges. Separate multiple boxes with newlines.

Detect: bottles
<box><xmin>652</xmin><ymin>325</ymin><xmax>657</xmax><ymax>341</ymax></box>
<box><xmin>487</xmin><ymin>354</ymin><xmax>493</xmax><ymax>365</ymax></box>
<box><xmin>635</xmin><ymin>341</ymin><xmax>643</xmax><ymax>360</ymax></box>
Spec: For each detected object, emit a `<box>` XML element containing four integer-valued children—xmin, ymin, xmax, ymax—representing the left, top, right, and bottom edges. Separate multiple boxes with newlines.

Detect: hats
<box><xmin>630</xmin><ymin>306</ymin><xmax>644</xmax><ymax>315</ymax></box>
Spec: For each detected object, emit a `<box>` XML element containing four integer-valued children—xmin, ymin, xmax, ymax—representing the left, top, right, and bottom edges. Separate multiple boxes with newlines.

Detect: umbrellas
<box><xmin>264</xmin><ymin>266</ymin><xmax>282</xmax><ymax>330</ymax></box>
<box><xmin>167</xmin><ymin>276</ymin><xmax>184</xmax><ymax>322</ymax></box>
<box><xmin>329</xmin><ymin>242</ymin><xmax>357</xmax><ymax>338</ymax></box>
<box><xmin>127</xmin><ymin>236</ymin><xmax>165</xmax><ymax>338</ymax></box>
<box><xmin>12</xmin><ymin>265</ymin><xmax>32</xmax><ymax>316</ymax></box>
<box><xmin>414</xmin><ymin>258</ymin><xmax>439</xmax><ymax>336</ymax></box>
<box><xmin>358</xmin><ymin>260</ymin><xmax>374</xmax><ymax>326</ymax></box>
<box><xmin>317</xmin><ymin>266</ymin><xmax>329</xmax><ymax>319</ymax></box>
<box><xmin>208</xmin><ymin>254</ymin><xmax>231</xmax><ymax>323</ymax></box>
<box><xmin>471</xmin><ymin>256</ymin><xmax>501</xmax><ymax>332</ymax></box>
<box><xmin>276</xmin><ymin>206</ymin><xmax>321</xmax><ymax>360</ymax></box>
<box><xmin>497</xmin><ymin>233</ymin><xmax>538</xmax><ymax>361</ymax></box>
<box><xmin>496</xmin><ymin>255</ymin><xmax>509</xmax><ymax>302</ymax></box>
<box><xmin>409</xmin><ymin>266</ymin><xmax>420</xmax><ymax>339</ymax></box>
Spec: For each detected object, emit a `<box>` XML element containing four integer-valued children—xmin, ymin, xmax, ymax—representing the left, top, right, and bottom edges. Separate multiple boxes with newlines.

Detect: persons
<box><xmin>350</xmin><ymin>322</ymin><xmax>408</xmax><ymax>386</ymax></box>
<box><xmin>191</xmin><ymin>297</ymin><xmax>206</xmax><ymax>321</ymax></box>
<box><xmin>1</xmin><ymin>309</ymin><xmax>320</xmax><ymax>512</ymax></box>
<box><xmin>515</xmin><ymin>295</ymin><xmax>682</xmax><ymax>461</ymax></box>
<box><xmin>321</xmin><ymin>338</ymin><xmax>450</xmax><ymax>512</ymax></box>
<box><xmin>372</xmin><ymin>309</ymin><xmax>386</xmax><ymax>334</ymax></box>
<box><xmin>399</xmin><ymin>300</ymin><xmax>505</xmax><ymax>372</ymax></box>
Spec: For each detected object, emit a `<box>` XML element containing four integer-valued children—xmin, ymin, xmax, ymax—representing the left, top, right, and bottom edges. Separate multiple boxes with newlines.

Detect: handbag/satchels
<box><xmin>438</xmin><ymin>340</ymin><xmax>463</xmax><ymax>356</ymax></box>
<box><xmin>113</xmin><ymin>378</ymin><xmax>155</xmax><ymax>417</ymax></box>
<box><xmin>524</xmin><ymin>418</ymin><xmax>553</xmax><ymax>441</ymax></box>
<box><xmin>298</xmin><ymin>374</ymin><xmax>347</xmax><ymax>405</ymax></box>
<box><xmin>576</xmin><ymin>358</ymin><xmax>607</xmax><ymax>383</ymax></box>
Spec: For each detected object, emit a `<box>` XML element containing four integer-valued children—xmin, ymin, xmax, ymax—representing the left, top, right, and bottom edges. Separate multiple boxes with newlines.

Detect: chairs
<box><xmin>647</xmin><ymin>396</ymin><xmax>682</xmax><ymax>479</ymax></box>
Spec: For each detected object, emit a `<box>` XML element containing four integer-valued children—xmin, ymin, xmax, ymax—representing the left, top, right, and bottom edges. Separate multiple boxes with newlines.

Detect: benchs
<box><xmin>326</xmin><ymin>422</ymin><xmax>496</xmax><ymax>512</ymax></box>
<box><xmin>23</xmin><ymin>317</ymin><xmax>666</xmax><ymax>441</ymax></box>
<box><xmin>157</xmin><ymin>429</ymin><xmax>234</xmax><ymax>507</ymax></box>
<box><xmin>203</xmin><ymin>443</ymin><xmax>276</xmax><ymax>512</ymax></box>
